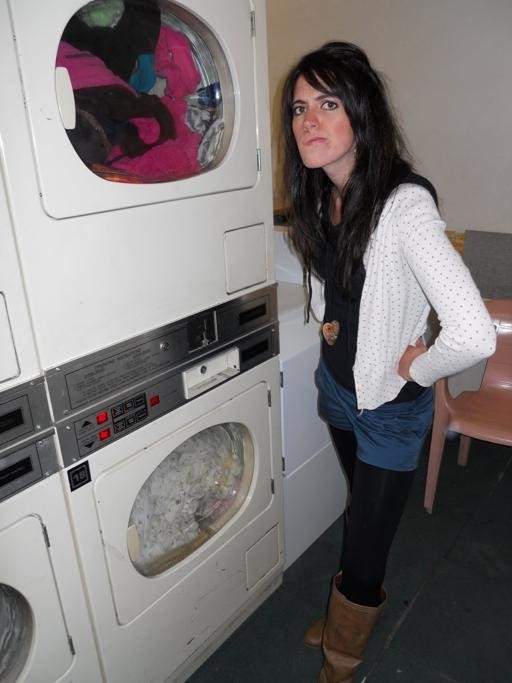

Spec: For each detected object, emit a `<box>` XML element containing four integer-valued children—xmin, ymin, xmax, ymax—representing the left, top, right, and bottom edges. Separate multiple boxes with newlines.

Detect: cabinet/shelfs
<box><xmin>279</xmin><ymin>341</ymin><xmax>352</xmax><ymax>573</ymax></box>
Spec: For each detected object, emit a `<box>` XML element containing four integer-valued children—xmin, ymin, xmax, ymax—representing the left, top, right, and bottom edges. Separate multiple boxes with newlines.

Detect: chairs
<box><xmin>421</xmin><ymin>293</ymin><xmax>511</xmax><ymax>517</ymax></box>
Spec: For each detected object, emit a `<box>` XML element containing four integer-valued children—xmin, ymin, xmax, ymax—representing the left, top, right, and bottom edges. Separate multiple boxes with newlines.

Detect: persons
<box><xmin>281</xmin><ymin>39</ymin><xmax>498</xmax><ymax>683</ymax></box>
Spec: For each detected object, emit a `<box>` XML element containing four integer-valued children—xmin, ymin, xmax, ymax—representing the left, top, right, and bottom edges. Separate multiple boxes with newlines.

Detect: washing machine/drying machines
<box><xmin>0</xmin><ymin>428</ymin><xmax>108</xmax><ymax>683</ymax></box>
<box><xmin>57</xmin><ymin>321</ymin><xmax>285</xmax><ymax>683</ymax></box>
<box><xmin>0</xmin><ymin>1</ymin><xmax>277</xmax><ymax>373</ymax></box>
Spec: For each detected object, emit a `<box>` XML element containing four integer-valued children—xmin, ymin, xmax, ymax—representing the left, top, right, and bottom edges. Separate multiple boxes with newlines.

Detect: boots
<box><xmin>303</xmin><ymin>614</ymin><xmax>326</xmax><ymax>650</ymax></box>
<box><xmin>317</xmin><ymin>570</ymin><xmax>389</xmax><ymax>681</ymax></box>
